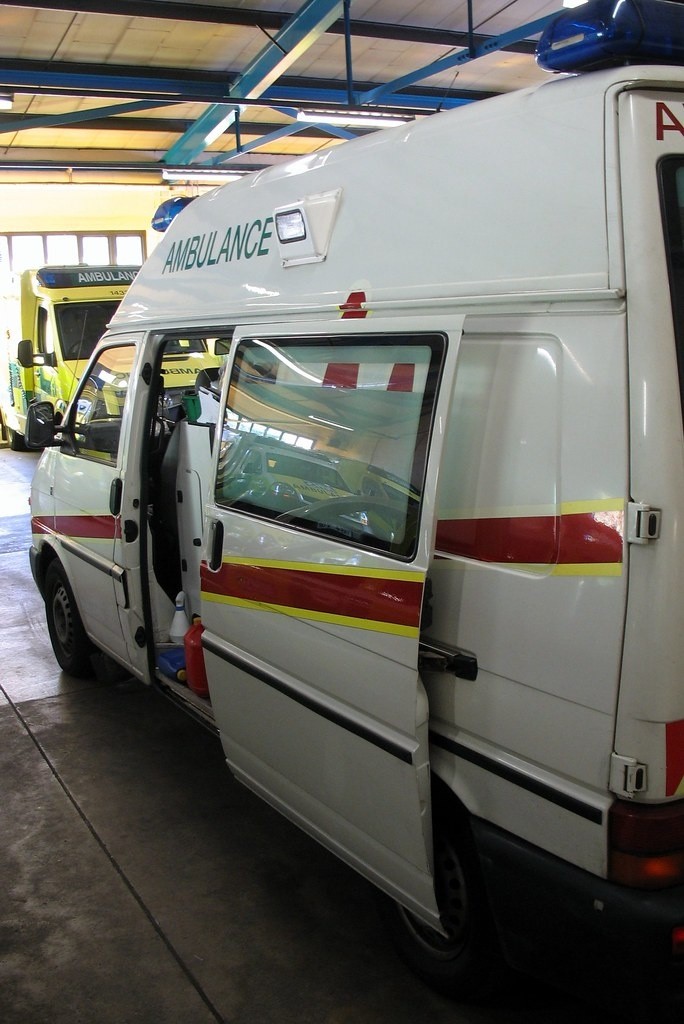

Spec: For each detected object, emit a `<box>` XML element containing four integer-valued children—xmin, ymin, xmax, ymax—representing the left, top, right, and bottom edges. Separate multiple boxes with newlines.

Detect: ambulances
<box><xmin>0</xmin><ymin>265</ymin><xmax>234</xmax><ymax>451</ymax></box>
<box><xmin>29</xmin><ymin>0</ymin><xmax>684</xmax><ymax>1024</ymax></box>
